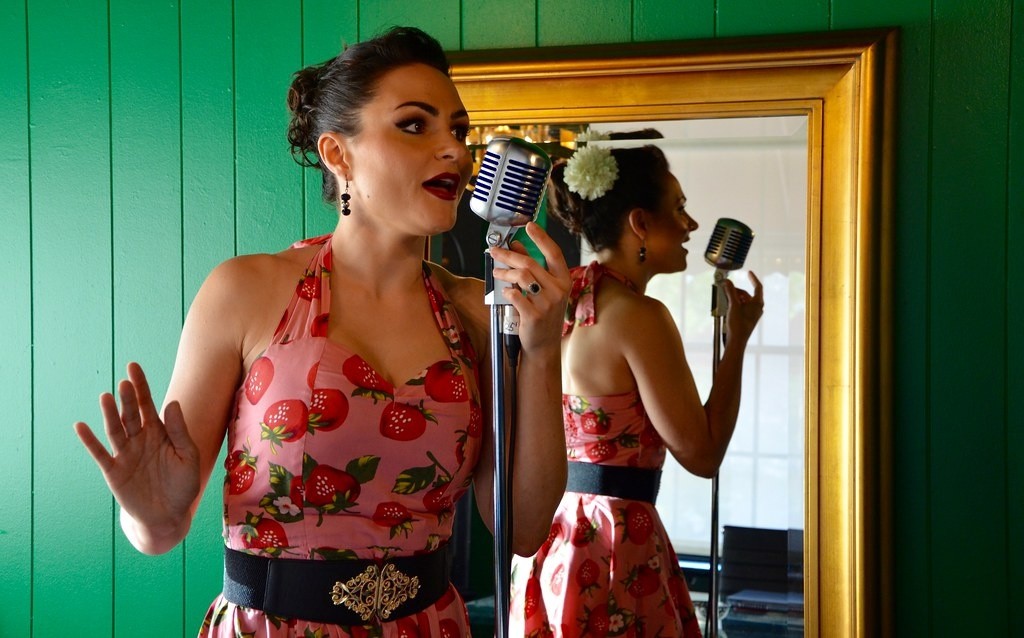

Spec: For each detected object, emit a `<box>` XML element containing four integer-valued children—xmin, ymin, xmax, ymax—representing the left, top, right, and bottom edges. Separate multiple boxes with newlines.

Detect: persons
<box><xmin>509</xmin><ymin>145</ymin><xmax>766</xmax><ymax>638</ymax></box>
<box><xmin>72</xmin><ymin>27</ymin><xmax>574</xmax><ymax>638</ymax></box>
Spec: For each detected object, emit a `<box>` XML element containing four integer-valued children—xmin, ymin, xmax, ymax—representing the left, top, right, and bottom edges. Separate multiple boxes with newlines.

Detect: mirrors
<box><xmin>424</xmin><ymin>25</ymin><xmax>897</xmax><ymax>637</ymax></box>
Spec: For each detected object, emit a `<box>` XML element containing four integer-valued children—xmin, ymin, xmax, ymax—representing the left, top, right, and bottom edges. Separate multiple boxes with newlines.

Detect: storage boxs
<box><xmin>720</xmin><ymin>606</ymin><xmax>804</xmax><ymax>638</ymax></box>
<box><xmin>721</xmin><ymin>524</ymin><xmax>804</xmax><ymax>609</ymax></box>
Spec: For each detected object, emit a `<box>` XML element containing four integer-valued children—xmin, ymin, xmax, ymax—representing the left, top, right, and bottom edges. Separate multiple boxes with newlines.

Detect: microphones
<box><xmin>704</xmin><ymin>218</ymin><xmax>755</xmax><ymax>346</ymax></box>
<box><xmin>470</xmin><ymin>135</ymin><xmax>552</xmax><ymax>366</ymax></box>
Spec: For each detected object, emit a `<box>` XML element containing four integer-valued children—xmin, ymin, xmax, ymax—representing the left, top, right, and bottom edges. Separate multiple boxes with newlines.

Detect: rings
<box><xmin>526</xmin><ymin>281</ymin><xmax>542</xmax><ymax>295</ymax></box>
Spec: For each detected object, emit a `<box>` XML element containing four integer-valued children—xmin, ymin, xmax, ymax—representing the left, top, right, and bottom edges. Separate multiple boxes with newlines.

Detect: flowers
<box><xmin>563</xmin><ymin>146</ymin><xmax>619</xmax><ymax>202</ymax></box>
